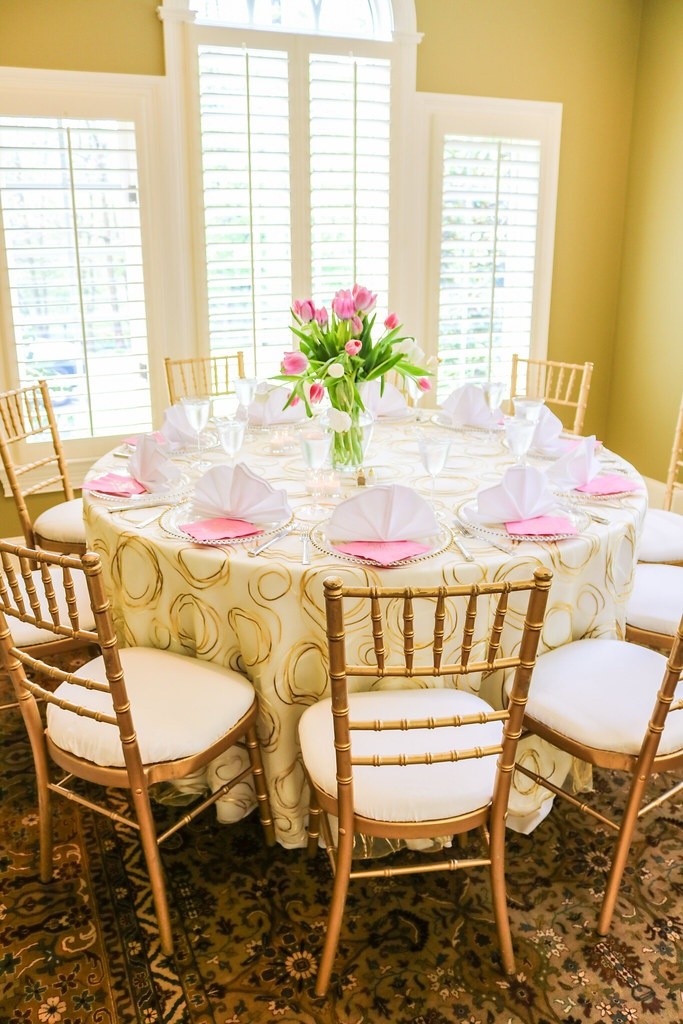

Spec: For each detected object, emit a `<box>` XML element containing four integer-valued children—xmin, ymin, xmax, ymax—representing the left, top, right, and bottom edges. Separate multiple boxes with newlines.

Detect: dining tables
<box><xmin>83</xmin><ymin>408</ymin><xmax>648</xmax><ymax>859</ymax></box>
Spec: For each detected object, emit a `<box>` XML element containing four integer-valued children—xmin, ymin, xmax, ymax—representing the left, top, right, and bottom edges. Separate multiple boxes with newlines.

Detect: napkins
<box><xmin>547</xmin><ymin>435</ymin><xmax>601</xmax><ymax>491</ymax></box>
<box><xmin>442</xmin><ymin>384</ymin><xmax>505</xmax><ymax>430</ymax></box>
<box><xmin>477</xmin><ymin>466</ymin><xmax>557</xmax><ymax>523</ymax></box>
<box><xmin>531</xmin><ymin>406</ymin><xmax>563</xmax><ymax>448</ymax></box>
<box><xmin>126</xmin><ymin>434</ymin><xmax>180</xmax><ymax>494</ymax></box>
<box><xmin>359</xmin><ymin>380</ymin><xmax>407</xmax><ymax>414</ymax></box>
<box><xmin>328</xmin><ymin>484</ymin><xmax>440</xmax><ymax>541</ymax></box>
<box><xmin>189</xmin><ymin>462</ymin><xmax>291</xmax><ymax>524</ymax></box>
<box><xmin>237</xmin><ymin>382</ymin><xmax>307</xmax><ymax>424</ymax></box>
<box><xmin>162</xmin><ymin>397</ymin><xmax>197</xmax><ymax>445</ymax></box>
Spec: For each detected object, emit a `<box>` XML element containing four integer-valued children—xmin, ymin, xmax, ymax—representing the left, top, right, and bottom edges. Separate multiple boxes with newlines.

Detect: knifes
<box><xmin>440</xmin><ymin>522</ymin><xmax>474</xmax><ymax>562</ymax></box>
<box><xmin>106</xmin><ymin>500</ymin><xmax>167</xmax><ymax>512</ymax></box>
<box><xmin>248</xmin><ymin>520</ymin><xmax>301</xmax><ymax>556</ymax></box>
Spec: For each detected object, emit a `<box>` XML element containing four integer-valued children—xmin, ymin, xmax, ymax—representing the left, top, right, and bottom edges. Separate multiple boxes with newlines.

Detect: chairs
<box><xmin>0</xmin><ymin>351</ymin><xmax>683</xmax><ymax>996</ymax></box>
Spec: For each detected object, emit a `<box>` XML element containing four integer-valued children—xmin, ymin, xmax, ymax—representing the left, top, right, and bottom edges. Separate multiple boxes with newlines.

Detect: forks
<box><xmin>454</xmin><ymin>520</ymin><xmax>517</xmax><ymax>556</ymax></box>
<box><xmin>301</xmin><ymin>523</ymin><xmax>310</xmax><ymax>564</ymax></box>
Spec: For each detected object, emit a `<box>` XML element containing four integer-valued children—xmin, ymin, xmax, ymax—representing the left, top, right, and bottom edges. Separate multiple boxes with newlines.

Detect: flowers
<box><xmin>267</xmin><ymin>282</ymin><xmax>432</xmax><ymax>465</ymax></box>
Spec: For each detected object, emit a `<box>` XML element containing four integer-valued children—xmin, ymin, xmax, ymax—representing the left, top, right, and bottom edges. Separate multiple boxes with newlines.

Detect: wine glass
<box><xmin>408</xmin><ymin>368</ymin><xmax>429</xmax><ymax>409</ymax></box>
<box><xmin>234</xmin><ymin>377</ymin><xmax>258</xmax><ymax>442</ymax></box>
<box><xmin>218</xmin><ymin>421</ymin><xmax>243</xmax><ymax>468</ymax></box>
<box><xmin>502</xmin><ymin>417</ymin><xmax>540</xmax><ymax>466</ymax></box>
<box><xmin>419</xmin><ymin>440</ymin><xmax>452</xmax><ymax>505</ymax></box>
<box><xmin>511</xmin><ymin>396</ymin><xmax>545</xmax><ymax>418</ymax></box>
<box><xmin>179</xmin><ymin>396</ymin><xmax>213</xmax><ymax>469</ymax></box>
<box><xmin>293</xmin><ymin>423</ymin><xmax>336</xmax><ymax>521</ymax></box>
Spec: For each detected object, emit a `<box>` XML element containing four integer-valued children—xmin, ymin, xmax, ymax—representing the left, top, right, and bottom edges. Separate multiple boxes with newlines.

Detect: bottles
<box><xmin>357</xmin><ymin>468</ymin><xmax>375</xmax><ymax>486</ymax></box>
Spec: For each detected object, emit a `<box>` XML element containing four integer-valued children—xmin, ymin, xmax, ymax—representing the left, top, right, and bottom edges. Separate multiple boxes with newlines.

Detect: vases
<box><xmin>321</xmin><ymin>406</ymin><xmax>374</xmax><ymax>471</ymax></box>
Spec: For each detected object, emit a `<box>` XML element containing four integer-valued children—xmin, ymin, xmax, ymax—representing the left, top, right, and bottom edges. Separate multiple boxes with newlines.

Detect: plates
<box><xmin>247</xmin><ymin>417</ymin><xmax>309</xmax><ymax>431</ymax></box>
<box><xmin>127</xmin><ymin>430</ymin><xmax>221</xmax><ymax>453</ymax></box>
<box><xmin>158</xmin><ymin>501</ymin><xmax>295</xmax><ymax>544</ymax></box>
<box><xmin>90</xmin><ymin>482</ymin><xmax>196</xmax><ymax>503</ymax></box>
<box><xmin>430</xmin><ymin>414</ymin><xmax>509</xmax><ymax>432</ymax></box>
<box><xmin>549</xmin><ymin>478</ymin><xmax>634</xmax><ymax>499</ymax></box>
<box><xmin>310</xmin><ymin>516</ymin><xmax>454</xmax><ymax>566</ymax></box>
<box><xmin>456</xmin><ymin>498</ymin><xmax>592</xmax><ymax>541</ymax></box>
<box><xmin>373</xmin><ymin>406</ymin><xmax>415</xmax><ymax>421</ymax></box>
<box><xmin>90</xmin><ymin>470</ymin><xmax>195</xmax><ymax>503</ymax></box>
